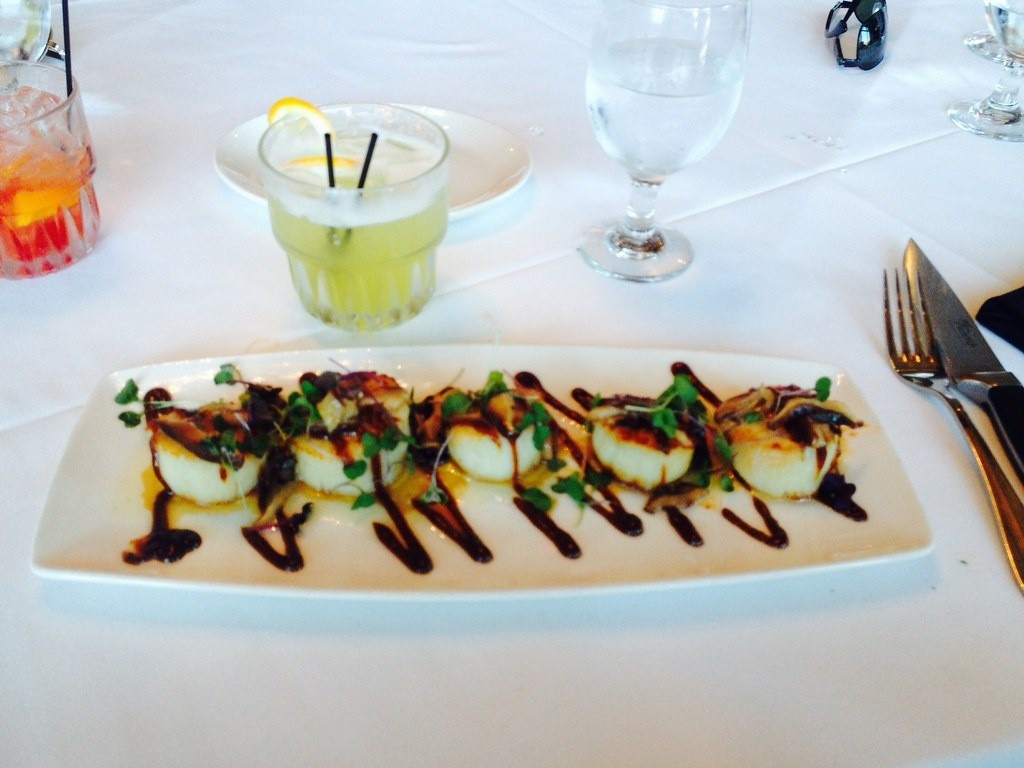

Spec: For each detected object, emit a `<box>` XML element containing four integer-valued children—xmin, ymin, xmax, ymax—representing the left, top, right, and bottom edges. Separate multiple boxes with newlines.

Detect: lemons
<box><xmin>283</xmin><ymin>156</ymin><xmax>363</xmax><ymax>175</ymax></box>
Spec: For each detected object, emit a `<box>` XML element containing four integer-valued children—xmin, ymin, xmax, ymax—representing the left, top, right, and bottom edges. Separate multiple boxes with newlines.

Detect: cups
<box><xmin>0</xmin><ymin>61</ymin><xmax>104</xmax><ymax>281</ymax></box>
<box><xmin>259</xmin><ymin>103</ymin><xmax>452</xmax><ymax>330</ymax></box>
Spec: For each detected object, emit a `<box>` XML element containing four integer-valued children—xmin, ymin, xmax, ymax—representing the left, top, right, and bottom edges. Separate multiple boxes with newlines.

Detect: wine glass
<box><xmin>944</xmin><ymin>0</ymin><xmax>1024</xmax><ymax>143</ymax></box>
<box><xmin>584</xmin><ymin>3</ymin><xmax>747</xmax><ymax>282</ymax></box>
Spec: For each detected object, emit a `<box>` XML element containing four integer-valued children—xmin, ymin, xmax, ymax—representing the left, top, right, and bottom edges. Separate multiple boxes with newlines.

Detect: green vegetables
<box><xmin>113</xmin><ymin>367</ymin><xmax>833</xmax><ymax>511</ymax></box>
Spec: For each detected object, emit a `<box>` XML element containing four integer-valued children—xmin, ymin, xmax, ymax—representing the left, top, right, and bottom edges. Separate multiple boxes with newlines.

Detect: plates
<box><xmin>211</xmin><ymin>102</ymin><xmax>533</xmax><ymax>221</ymax></box>
<box><xmin>27</xmin><ymin>344</ymin><xmax>936</xmax><ymax>603</ymax></box>
<box><xmin>0</xmin><ymin>0</ymin><xmax>52</xmax><ymax>62</ymax></box>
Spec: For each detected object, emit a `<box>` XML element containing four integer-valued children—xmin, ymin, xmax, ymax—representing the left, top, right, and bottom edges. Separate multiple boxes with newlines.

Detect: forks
<box><xmin>882</xmin><ymin>269</ymin><xmax>1024</xmax><ymax>595</ymax></box>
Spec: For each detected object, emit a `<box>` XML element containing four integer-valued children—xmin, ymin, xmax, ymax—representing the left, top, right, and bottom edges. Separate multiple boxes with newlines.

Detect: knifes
<box><xmin>899</xmin><ymin>235</ymin><xmax>1024</xmax><ymax>503</ymax></box>
